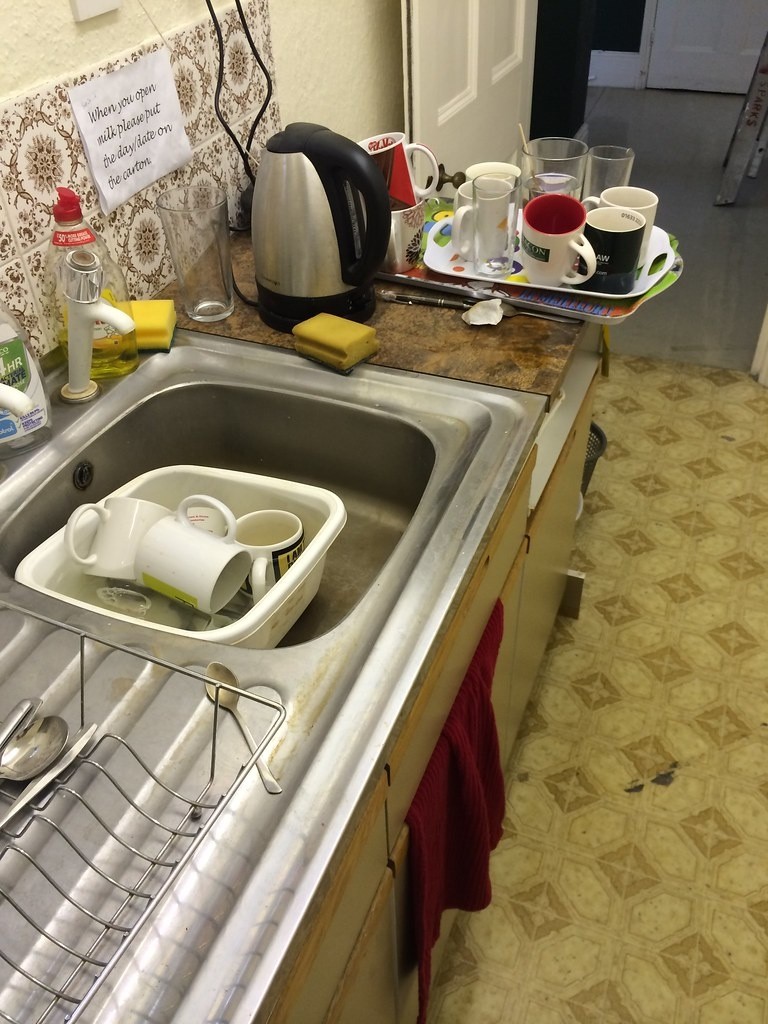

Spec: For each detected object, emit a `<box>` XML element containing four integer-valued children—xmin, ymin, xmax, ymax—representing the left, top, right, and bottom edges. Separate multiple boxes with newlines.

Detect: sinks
<box><xmin>0</xmin><ymin>328</ymin><xmax>547</xmax><ymax>682</ymax></box>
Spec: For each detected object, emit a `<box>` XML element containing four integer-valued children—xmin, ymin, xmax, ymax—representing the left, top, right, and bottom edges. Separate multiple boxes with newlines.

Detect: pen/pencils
<box><xmin>380</xmin><ymin>289</ymin><xmax>473</xmax><ymax>310</ymax></box>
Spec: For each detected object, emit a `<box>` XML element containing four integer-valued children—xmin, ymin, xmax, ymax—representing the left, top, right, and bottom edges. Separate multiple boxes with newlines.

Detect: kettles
<box><xmin>251</xmin><ymin>123</ymin><xmax>389</xmax><ymax>336</ymax></box>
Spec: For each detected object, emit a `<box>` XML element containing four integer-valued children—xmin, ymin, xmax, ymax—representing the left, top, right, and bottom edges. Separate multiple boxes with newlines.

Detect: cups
<box><xmin>473</xmin><ymin>173</ymin><xmax>519</xmax><ymax>280</ymax></box>
<box><xmin>583</xmin><ymin>207</ymin><xmax>646</xmax><ymax>294</ymax></box>
<box><xmin>174</xmin><ymin>506</ymin><xmax>229</xmax><ymax>537</ymax></box>
<box><xmin>156</xmin><ymin>186</ymin><xmax>234</xmax><ymax>322</ymax></box>
<box><xmin>519</xmin><ymin>194</ymin><xmax>596</xmax><ymax>288</ymax></box>
<box><xmin>134</xmin><ymin>495</ymin><xmax>252</xmax><ymax>614</ymax></box>
<box><xmin>522</xmin><ymin>137</ymin><xmax>588</xmax><ymax>197</ymax></box>
<box><xmin>384</xmin><ymin>197</ymin><xmax>424</xmax><ymax>274</ymax></box>
<box><xmin>466</xmin><ymin>162</ymin><xmax>522</xmax><ymax>183</ymax></box>
<box><xmin>237</xmin><ymin>510</ymin><xmax>304</xmax><ymax>605</ymax></box>
<box><xmin>581</xmin><ymin>186</ymin><xmax>659</xmax><ymax>270</ymax></box>
<box><xmin>65</xmin><ymin>497</ymin><xmax>170</xmax><ymax>580</ymax></box>
<box><xmin>353</xmin><ymin>132</ymin><xmax>439</xmax><ymax>210</ymax></box>
<box><xmin>451</xmin><ymin>180</ymin><xmax>513</xmax><ymax>262</ymax></box>
<box><xmin>582</xmin><ymin>147</ymin><xmax>635</xmax><ymax>194</ymax></box>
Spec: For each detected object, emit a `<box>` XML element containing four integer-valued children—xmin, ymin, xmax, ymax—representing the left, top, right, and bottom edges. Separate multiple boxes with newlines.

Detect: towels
<box><xmin>400</xmin><ymin>598</ymin><xmax>508</xmax><ymax>1024</ymax></box>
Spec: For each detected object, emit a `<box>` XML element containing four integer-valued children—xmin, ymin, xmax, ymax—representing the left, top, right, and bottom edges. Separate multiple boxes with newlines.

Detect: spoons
<box><xmin>0</xmin><ymin>716</ymin><xmax>69</xmax><ymax>781</ymax></box>
<box><xmin>206</xmin><ymin>662</ymin><xmax>283</xmax><ymax>793</ymax></box>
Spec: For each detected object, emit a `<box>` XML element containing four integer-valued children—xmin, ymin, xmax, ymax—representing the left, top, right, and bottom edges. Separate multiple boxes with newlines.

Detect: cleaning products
<box><xmin>41</xmin><ymin>186</ymin><xmax>141</xmax><ymax>379</ymax></box>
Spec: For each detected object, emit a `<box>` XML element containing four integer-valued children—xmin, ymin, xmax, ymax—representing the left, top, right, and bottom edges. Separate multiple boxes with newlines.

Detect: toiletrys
<box><xmin>0</xmin><ymin>298</ymin><xmax>53</xmax><ymax>461</ymax></box>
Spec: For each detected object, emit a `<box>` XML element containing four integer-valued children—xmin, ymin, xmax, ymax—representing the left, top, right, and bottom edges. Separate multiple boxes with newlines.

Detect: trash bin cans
<box><xmin>581</xmin><ymin>421</ymin><xmax>607</xmax><ymax>495</ymax></box>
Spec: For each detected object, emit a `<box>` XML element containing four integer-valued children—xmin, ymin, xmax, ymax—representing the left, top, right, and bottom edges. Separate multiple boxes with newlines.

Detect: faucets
<box><xmin>59</xmin><ymin>249</ymin><xmax>138</xmax><ymax>403</ymax></box>
<box><xmin>0</xmin><ymin>383</ymin><xmax>33</xmax><ymax>419</ymax></box>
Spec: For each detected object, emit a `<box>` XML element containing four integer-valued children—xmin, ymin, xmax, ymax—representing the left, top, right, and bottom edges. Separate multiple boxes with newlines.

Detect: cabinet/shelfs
<box><xmin>255</xmin><ymin>324</ymin><xmax>601</xmax><ymax>1024</ymax></box>
